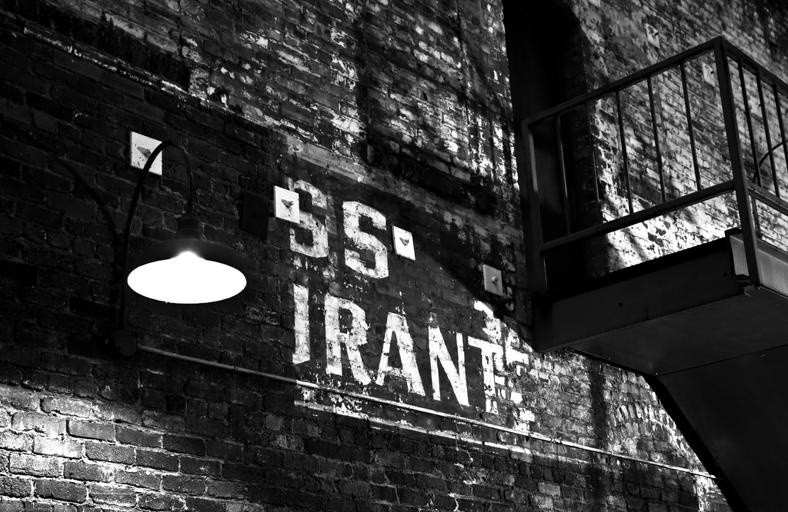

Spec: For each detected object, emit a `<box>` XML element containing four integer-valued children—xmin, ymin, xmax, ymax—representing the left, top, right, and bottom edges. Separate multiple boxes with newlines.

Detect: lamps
<box><xmin>115</xmin><ymin>138</ymin><xmax>249</xmax><ymax>331</ymax></box>
